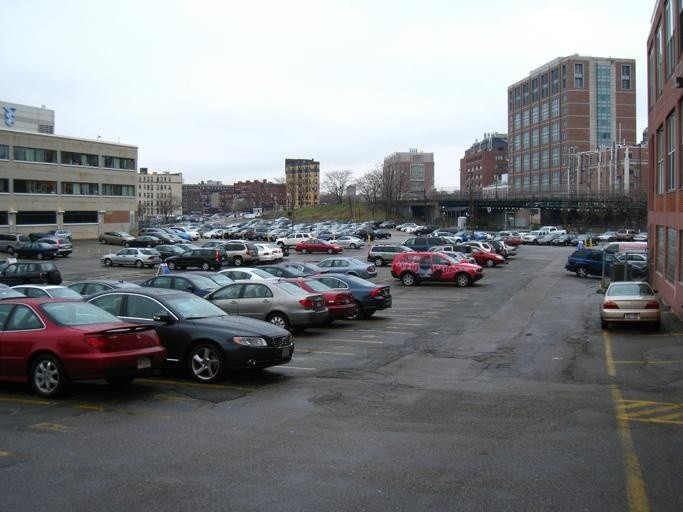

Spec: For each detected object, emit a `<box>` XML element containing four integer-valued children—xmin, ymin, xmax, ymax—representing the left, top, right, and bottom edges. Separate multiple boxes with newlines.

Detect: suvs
<box><xmin>366</xmin><ymin>243</ymin><xmax>417</xmax><ymax>267</ymax></box>
<box><xmin>0</xmin><ymin>259</ymin><xmax>63</xmax><ymax>287</ymax></box>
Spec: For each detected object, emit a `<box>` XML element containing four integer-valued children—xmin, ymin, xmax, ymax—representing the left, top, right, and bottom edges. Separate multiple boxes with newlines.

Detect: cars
<box><xmin>98</xmin><ymin>230</ymin><xmax>137</xmax><ymax>246</ymax></box>
<box><xmin>9</xmin><ymin>283</ymin><xmax>84</xmax><ymax>300</ymax></box>
<box><xmin>134</xmin><ymin>211</ymin><xmax>398</xmax><ymax>272</ymax></box>
<box><xmin>316</xmin><ymin>256</ymin><xmax>378</xmax><ymax>280</ymax></box>
<box><xmin>66</xmin><ymin>278</ymin><xmax>142</xmax><ymax>298</ymax></box>
<box><xmin>201</xmin><ymin>260</ymin><xmax>391</xmax><ymax>337</ymax></box>
<box><xmin>124</xmin><ymin>235</ymin><xmax>165</xmax><ymax>248</ymax></box>
<box><xmin>0</xmin><ymin>296</ymin><xmax>167</xmax><ymax>398</ymax></box>
<box><xmin>102</xmin><ymin>247</ymin><xmax>163</xmax><ymax>269</ymax></box>
<box><xmin>498</xmin><ymin>225</ymin><xmax>658</xmax><ymax>281</ymax></box>
<box><xmin>82</xmin><ymin>285</ymin><xmax>296</xmax><ymax>385</ymax></box>
<box><xmin>0</xmin><ymin>227</ymin><xmax>73</xmax><ymax>261</ymax></box>
<box><xmin>596</xmin><ymin>282</ymin><xmax>667</xmax><ymax>334</ymax></box>
<box><xmin>392</xmin><ymin>222</ymin><xmax>520</xmax><ymax>287</ymax></box>
<box><xmin>138</xmin><ymin>271</ymin><xmax>236</xmax><ymax>299</ymax></box>
<box><xmin>0</xmin><ymin>282</ymin><xmax>27</xmax><ymax>300</ymax></box>
